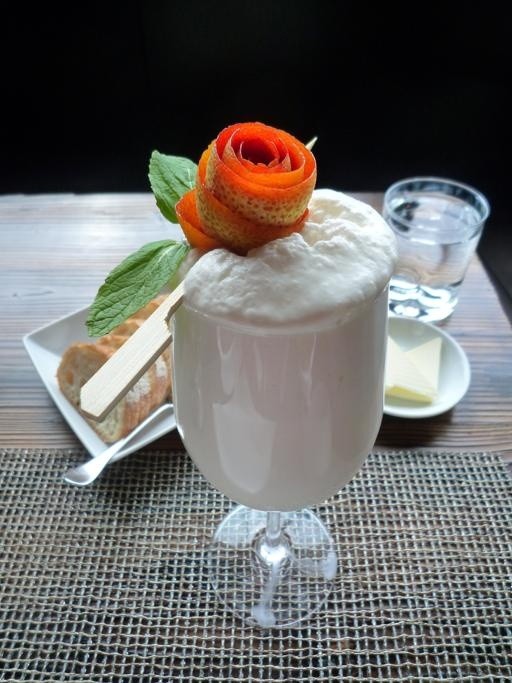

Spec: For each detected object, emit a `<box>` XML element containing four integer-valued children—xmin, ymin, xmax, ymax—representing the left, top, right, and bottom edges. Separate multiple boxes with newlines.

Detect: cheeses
<box><xmin>385</xmin><ymin>335</ymin><xmax>442</xmax><ymax>404</ymax></box>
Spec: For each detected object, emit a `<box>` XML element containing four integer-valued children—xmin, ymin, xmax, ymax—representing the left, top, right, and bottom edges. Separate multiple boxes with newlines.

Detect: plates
<box><xmin>379</xmin><ymin>314</ymin><xmax>472</xmax><ymax>422</ymax></box>
<box><xmin>21</xmin><ymin>283</ymin><xmax>175</xmax><ymax>467</ymax></box>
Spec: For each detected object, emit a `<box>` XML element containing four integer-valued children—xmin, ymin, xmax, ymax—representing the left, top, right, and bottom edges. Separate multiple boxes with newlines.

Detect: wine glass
<box><xmin>167</xmin><ymin>272</ymin><xmax>393</xmax><ymax>633</ymax></box>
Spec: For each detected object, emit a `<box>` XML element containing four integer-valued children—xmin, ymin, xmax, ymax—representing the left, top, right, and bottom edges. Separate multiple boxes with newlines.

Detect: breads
<box><xmin>55</xmin><ymin>291</ymin><xmax>175</xmax><ymax>444</ymax></box>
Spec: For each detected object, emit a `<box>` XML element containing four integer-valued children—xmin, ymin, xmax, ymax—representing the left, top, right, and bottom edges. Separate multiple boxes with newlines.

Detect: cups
<box><xmin>381</xmin><ymin>174</ymin><xmax>495</xmax><ymax>322</ymax></box>
